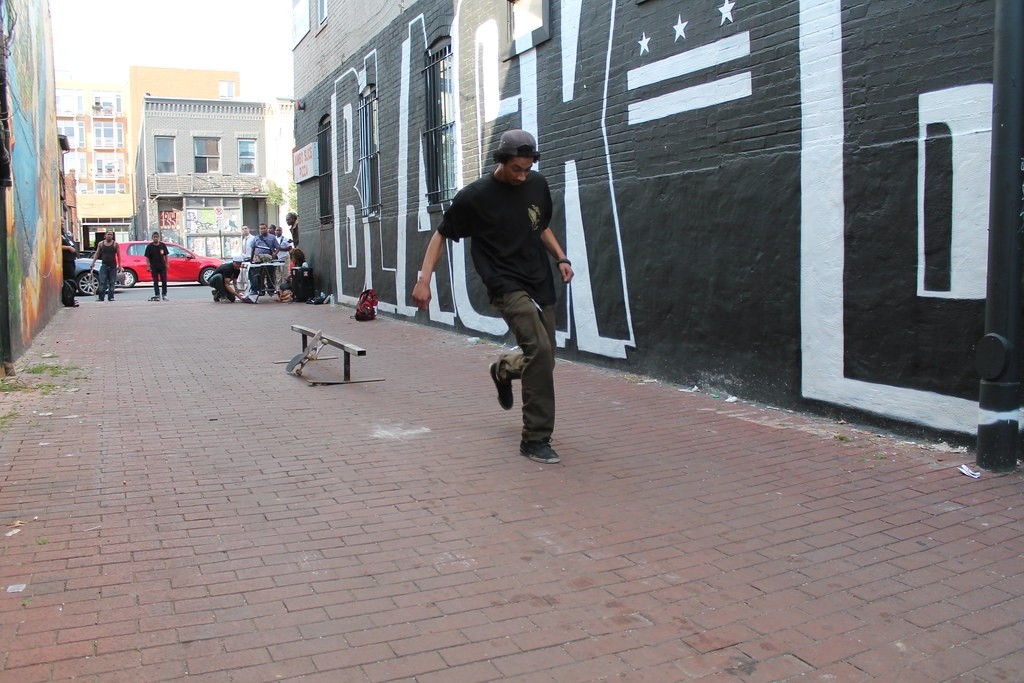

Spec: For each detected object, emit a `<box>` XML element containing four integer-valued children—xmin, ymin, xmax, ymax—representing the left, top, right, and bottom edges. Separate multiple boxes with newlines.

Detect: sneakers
<box><xmin>488</xmin><ymin>362</ymin><xmax>514</xmax><ymax>410</ymax></box>
<box><xmin>519</xmin><ymin>439</ymin><xmax>561</xmax><ymax>464</ymax></box>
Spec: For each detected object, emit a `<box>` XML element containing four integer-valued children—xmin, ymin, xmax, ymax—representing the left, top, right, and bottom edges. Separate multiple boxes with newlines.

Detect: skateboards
<box><xmin>286</xmin><ymin>330</ymin><xmax>330</xmax><ymax>375</ymax></box>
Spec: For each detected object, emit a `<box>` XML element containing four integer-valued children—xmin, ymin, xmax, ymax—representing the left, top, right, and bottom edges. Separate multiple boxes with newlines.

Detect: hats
<box><xmin>498</xmin><ymin>129</ymin><xmax>539</xmax><ymax>157</ymax></box>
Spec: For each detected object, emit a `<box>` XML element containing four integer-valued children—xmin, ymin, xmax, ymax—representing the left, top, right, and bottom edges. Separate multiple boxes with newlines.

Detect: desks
<box><xmin>240</xmin><ymin>261</ymin><xmax>285</xmax><ymax>303</ymax></box>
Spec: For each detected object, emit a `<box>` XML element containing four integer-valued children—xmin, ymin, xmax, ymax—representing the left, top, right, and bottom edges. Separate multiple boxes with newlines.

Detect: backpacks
<box><xmin>355</xmin><ymin>289</ymin><xmax>378</xmax><ymax>321</ymax></box>
<box><xmin>62</xmin><ymin>279</ymin><xmax>78</xmax><ymax>306</ymax></box>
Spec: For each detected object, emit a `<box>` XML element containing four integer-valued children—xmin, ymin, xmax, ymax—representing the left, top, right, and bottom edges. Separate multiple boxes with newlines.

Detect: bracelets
<box><xmin>557</xmin><ymin>259</ymin><xmax>571</xmax><ymax>266</ymax></box>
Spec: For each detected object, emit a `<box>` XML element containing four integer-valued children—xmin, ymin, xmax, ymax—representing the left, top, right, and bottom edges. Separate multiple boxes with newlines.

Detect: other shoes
<box><xmin>249</xmin><ymin>291</ymin><xmax>258</xmax><ymax>295</ymax></box>
<box><xmin>274</xmin><ymin>290</ymin><xmax>279</xmax><ymax>294</ymax></box>
<box><xmin>212</xmin><ymin>290</ymin><xmax>220</xmax><ymax>302</ymax></box>
<box><xmin>96</xmin><ymin>300</ymin><xmax>104</xmax><ymax>302</ymax></box>
<box><xmin>259</xmin><ymin>291</ymin><xmax>265</xmax><ymax>296</ymax></box>
<box><xmin>109</xmin><ymin>297</ymin><xmax>115</xmax><ymax>302</ymax></box>
<box><xmin>161</xmin><ymin>296</ymin><xmax>170</xmax><ymax>301</ymax></box>
<box><xmin>154</xmin><ymin>295</ymin><xmax>160</xmax><ymax>301</ymax></box>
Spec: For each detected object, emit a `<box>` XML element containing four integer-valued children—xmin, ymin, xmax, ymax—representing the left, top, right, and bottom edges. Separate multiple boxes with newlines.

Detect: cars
<box><xmin>114</xmin><ymin>240</ymin><xmax>225</xmax><ymax>288</ymax></box>
<box><xmin>75</xmin><ymin>257</ymin><xmax>103</xmax><ymax>295</ymax></box>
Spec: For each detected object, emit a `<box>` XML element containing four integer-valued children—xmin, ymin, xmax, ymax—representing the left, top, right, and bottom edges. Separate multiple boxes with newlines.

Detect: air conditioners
<box><xmin>93</xmin><ymin>101</ymin><xmax>103</xmax><ymax>108</ymax></box>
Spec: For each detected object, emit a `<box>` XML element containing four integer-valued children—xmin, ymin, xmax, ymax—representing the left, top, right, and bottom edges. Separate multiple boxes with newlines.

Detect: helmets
<box><xmin>233</xmin><ymin>254</ymin><xmax>244</xmax><ymax>262</ymax></box>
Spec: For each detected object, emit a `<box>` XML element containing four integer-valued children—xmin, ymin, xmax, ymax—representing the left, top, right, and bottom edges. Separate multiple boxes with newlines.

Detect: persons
<box><xmin>286</xmin><ymin>212</ymin><xmax>298</xmax><ymax>248</ymax></box>
<box><xmin>249</xmin><ymin>223</ymin><xmax>292</xmax><ymax>296</ymax></box>
<box><xmin>144</xmin><ymin>231</ymin><xmax>169</xmax><ymax>300</ymax></box>
<box><xmin>277</xmin><ymin>249</ymin><xmax>309</xmax><ymax>302</ymax></box>
<box><xmin>412</xmin><ymin>129</ymin><xmax>575</xmax><ymax>464</ymax></box>
<box><xmin>207</xmin><ymin>254</ymin><xmax>245</xmax><ymax>303</ymax></box>
<box><xmin>240</xmin><ymin>225</ymin><xmax>254</xmax><ymax>292</ymax></box>
<box><xmin>60</xmin><ymin>216</ymin><xmax>79</xmax><ymax>307</ymax></box>
<box><xmin>90</xmin><ymin>230</ymin><xmax>122</xmax><ymax>301</ymax></box>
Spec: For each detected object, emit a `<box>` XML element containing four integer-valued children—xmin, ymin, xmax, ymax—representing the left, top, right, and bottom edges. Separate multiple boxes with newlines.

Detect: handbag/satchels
<box><xmin>271</xmin><ymin>250</ymin><xmax>278</xmax><ymax>259</ymax></box>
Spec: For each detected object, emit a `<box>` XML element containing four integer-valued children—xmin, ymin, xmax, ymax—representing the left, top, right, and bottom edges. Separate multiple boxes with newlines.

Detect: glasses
<box><xmin>107</xmin><ymin>233</ymin><xmax>113</xmax><ymax>235</ymax></box>
<box><xmin>242</xmin><ymin>228</ymin><xmax>247</xmax><ymax>230</ymax></box>
